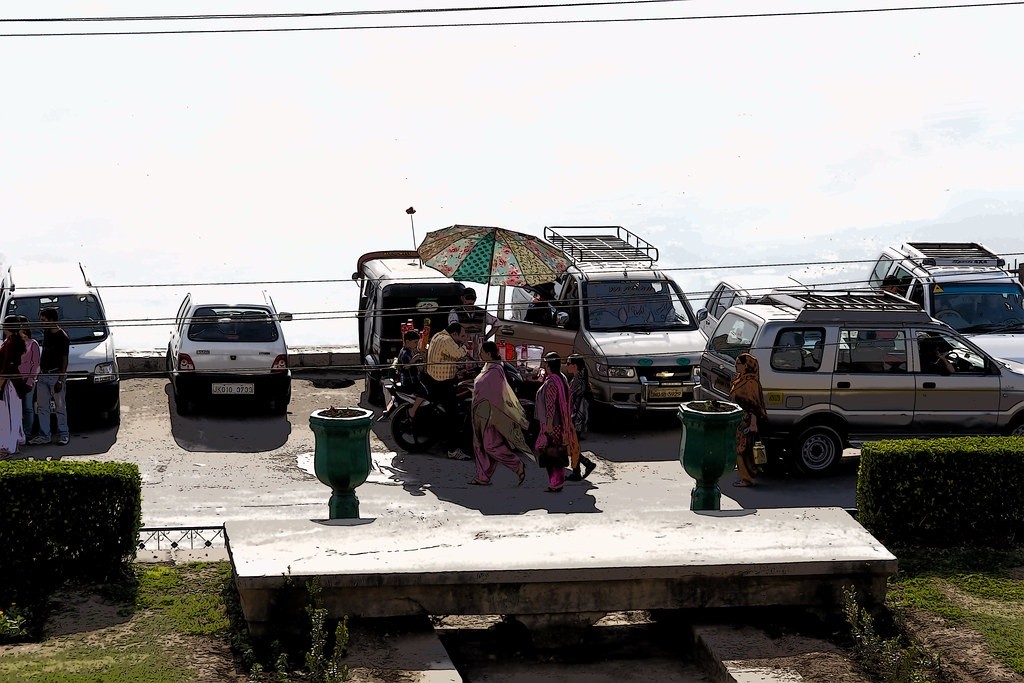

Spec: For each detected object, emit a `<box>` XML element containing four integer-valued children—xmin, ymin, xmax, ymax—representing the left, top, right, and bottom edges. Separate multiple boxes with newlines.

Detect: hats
<box><xmin>461</xmin><ymin>287</ymin><xmax>476</xmax><ymax>296</ymax></box>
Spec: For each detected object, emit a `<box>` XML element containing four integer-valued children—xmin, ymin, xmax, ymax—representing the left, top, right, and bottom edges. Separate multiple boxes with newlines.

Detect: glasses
<box><xmin>565</xmin><ymin>360</ymin><xmax>572</xmax><ymax>366</ymax></box>
<box><xmin>464</xmin><ymin>294</ymin><xmax>477</xmax><ymax>300</ymax></box>
<box><xmin>735</xmin><ymin>361</ymin><xmax>742</xmax><ymax>365</ymax></box>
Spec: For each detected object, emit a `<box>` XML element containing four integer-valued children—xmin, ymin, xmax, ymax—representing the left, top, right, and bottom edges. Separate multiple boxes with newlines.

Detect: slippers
<box><xmin>517</xmin><ymin>461</ymin><xmax>525</xmax><ymax>486</ymax></box>
<box><xmin>466</xmin><ymin>479</ymin><xmax>479</xmax><ymax>485</ymax></box>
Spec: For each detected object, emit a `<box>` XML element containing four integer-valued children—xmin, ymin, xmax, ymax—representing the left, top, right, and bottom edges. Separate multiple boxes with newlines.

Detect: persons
<box><xmin>524</xmin><ymin>282</ymin><xmax>556</xmax><ymax>325</ymax></box>
<box><xmin>467</xmin><ymin>341</ymin><xmax>537</xmax><ymax>488</ymax></box>
<box><xmin>17</xmin><ymin>316</ymin><xmax>40</xmax><ymax>434</ymax></box>
<box><xmin>396</xmin><ymin>330</ymin><xmax>427</xmax><ymax>424</ymax></box>
<box><xmin>565</xmin><ymin>354</ymin><xmax>597</xmax><ymax>482</ymax></box>
<box><xmin>919</xmin><ymin>337</ymin><xmax>955</xmax><ymax>376</ymax></box>
<box><xmin>534</xmin><ymin>351</ymin><xmax>572</xmax><ymax>493</ymax></box>
<box><xmin>728</xmin><ymin>353</ymin><xmax>769</xmax><ymax>490</ymax></box>
<box><xmin>29</xmin><ymin>308</ymin><xmax>70</xmax><ymax>445</ymax></box>
<box><xmin>881</xmin><ymin>275</ymin><xmax>900</xmax><ymax>294</ymax></box>
<box><xmin>448</xmin><ymin>288</ymin><xmax>501</xmax><ymax>341</ymax></box>
<box><xmin>0</xmin><ymin>315</ymin><xmax>26</xmax><ymax>455</ymax></box>
<box><xmin>427</xmin><ymin>323</ymin><xmax>470</xmax><ymax>461</ymax></box>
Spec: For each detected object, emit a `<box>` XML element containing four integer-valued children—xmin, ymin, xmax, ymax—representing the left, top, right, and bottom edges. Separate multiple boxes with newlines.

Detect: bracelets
<box><xmin>57</xmin><ymin>380</ymin><xmax>63</xmax><ymax>384</ymax></box>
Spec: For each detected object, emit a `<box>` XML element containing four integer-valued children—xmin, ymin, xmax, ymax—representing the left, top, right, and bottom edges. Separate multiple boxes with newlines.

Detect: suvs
<box><xmin>0</xmin><ymin>262</ymin><xmax>121</xmax><ymax>431</ymax></box>
<box><xmin>868</xmin><ymin>241</ymin><xmax>1024</xmax><ymax>365</ymax></box>
<box><xmin>166</xmin><ymin>292</ymin><xmax>293</xmax><ymax>417</ymax></box>
<box><xmin>700</xmin><ymin>273</ymin><xmax>814</xmax><ymax>338</ymax></box>
<box><xmin>693</xmin><ymin>288</ymin><xmax>1024</xmax><ymax>483</ymax></box>
<box><xmin>495</xmin><ymin>225</ymin><xmax>712</xmax><ymax>433</ymax></box>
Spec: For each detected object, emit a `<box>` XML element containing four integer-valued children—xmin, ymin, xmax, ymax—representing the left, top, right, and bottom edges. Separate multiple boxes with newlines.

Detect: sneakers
<box><xmin>448</xmin><ymin>448</ymin><xmax>471</xmax><ymax>461</ymax></box>
<box><xmin>59</xmin><ymin>436</ymin><xmax>70</xmax><ymax>444</ymax></box>
<box><xmin>28</xmin><ymin>436</ymin><xmax>51</xmax><ymax>445</ymax></box>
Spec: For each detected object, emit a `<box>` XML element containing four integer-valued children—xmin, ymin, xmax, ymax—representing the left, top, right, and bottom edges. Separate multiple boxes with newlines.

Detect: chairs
<box><xmin>789</xmin><ymin>332</ymin><xmax>805</xmax><ymax>368</ymax></box>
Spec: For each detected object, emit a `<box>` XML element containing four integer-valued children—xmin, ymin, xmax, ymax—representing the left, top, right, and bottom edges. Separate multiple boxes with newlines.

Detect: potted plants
<box><xmin>309</xmin><ymin>405</ymin><xmax>375</xmax><ymax>491</ymax></box>
<box><xmin>676</xmin><ymin>398</ymin><xmax>743</xmax><ymax>479</ymax></box>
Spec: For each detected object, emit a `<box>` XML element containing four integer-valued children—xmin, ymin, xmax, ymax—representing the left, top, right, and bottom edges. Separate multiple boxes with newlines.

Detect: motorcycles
<box><xmin>375</xmin><ymin>359</ymin><xmax>538</xmax><ymax>456</ymax></box>
<box><xmin>353</xmin><ymin>250</ymin><xmax>469</xmax><ymax>408</ymax></box>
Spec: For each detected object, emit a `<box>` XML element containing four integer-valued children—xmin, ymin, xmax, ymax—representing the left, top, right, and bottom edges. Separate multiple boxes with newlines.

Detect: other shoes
<box><xmin>565</xmin><ymin>473</ymin><xmax>582</xmax><ymax>481</ymax></box>
<box><xmin>734</xmin><ymin>478</ymin><xmax>757</xmax><ymax>487</ymax></box>
<box><xmin>406</xmin><ymin>408</ymin><xmax>416</xmax><ymax>424</ymax></box>
<box><xmin>545</xmin><ymin>485</ymin><xmax>565</xmax><ymax>492</ymax></box>
<box><xmin>583</xmin><ymin>462</ymin><xmax>596</xmax><ymax>477</ymax></box>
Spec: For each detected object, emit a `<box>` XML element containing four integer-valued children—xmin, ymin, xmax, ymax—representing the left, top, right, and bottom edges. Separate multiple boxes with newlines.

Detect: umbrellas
<box><xmin>417</xmin><ymin>224</ymin><xmax>572</xmax><ymax>310</ymax></box>
